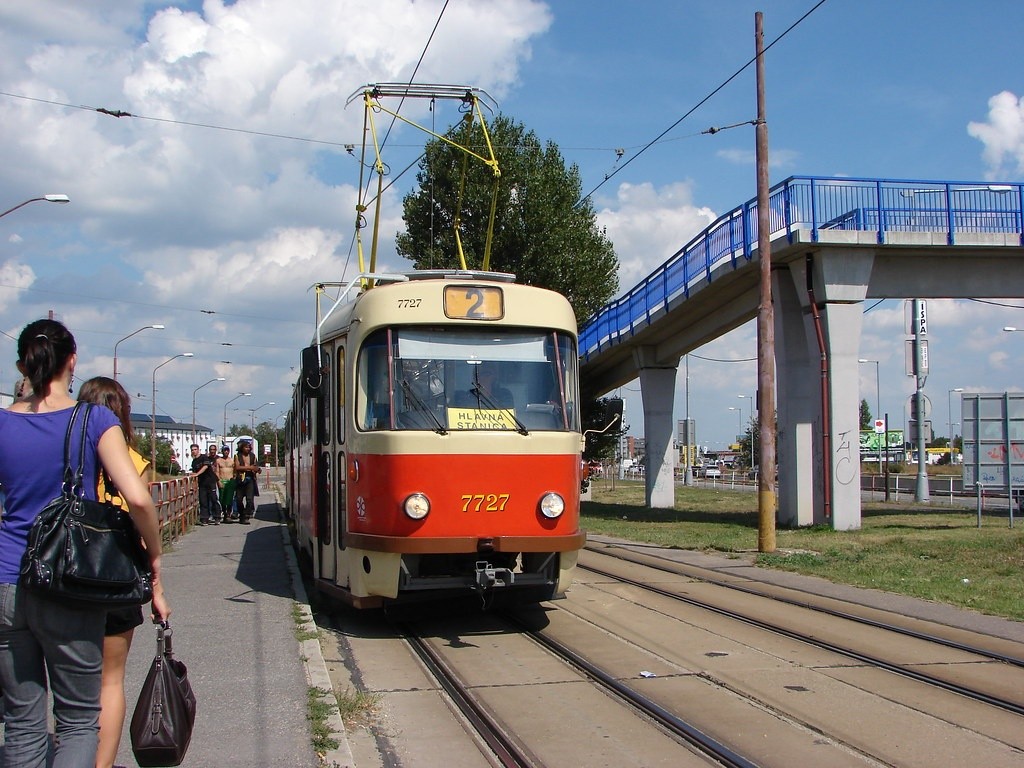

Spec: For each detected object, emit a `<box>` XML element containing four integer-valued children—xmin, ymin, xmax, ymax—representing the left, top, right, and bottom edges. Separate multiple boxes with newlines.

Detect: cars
<box><xmin>697</xmin><ymin>465</ymin><xmax>721</xmax><ymax>479</ymax></box>
<box><xmin>691</xmin><ymin>466</ymin><xmax>702</xmax><ymax>476</ymax></box>
<box><xmin>748</xmin><ymin>464</ymin><xmax>778</xmax><ymax>481</ymax></box>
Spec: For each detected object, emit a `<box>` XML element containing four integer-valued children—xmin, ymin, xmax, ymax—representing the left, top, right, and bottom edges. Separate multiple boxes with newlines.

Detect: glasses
<box><xmin>479</xmin><ymin>371</ymin><xmax>494</xmax><ymax>376</ymax></box>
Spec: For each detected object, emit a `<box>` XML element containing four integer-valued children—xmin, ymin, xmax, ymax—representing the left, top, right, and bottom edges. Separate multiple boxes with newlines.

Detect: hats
<box><xmin>222</xmin><ymin>445</ymin><xmax>229</xmax><ymax>449</ymax></box>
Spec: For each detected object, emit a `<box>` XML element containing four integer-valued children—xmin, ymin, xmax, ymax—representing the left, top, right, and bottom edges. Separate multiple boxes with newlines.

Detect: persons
<box><xmin>0</xmin><ymin>319</ymin><xmax>162</xmax><ymax>768</ymax></box>
<box><xmin>190</xmin><ymin>441</ymin><xmax>262</xmax><ymax>526</ymax></box>
<box><xmin>78</xmin><ymin>376</ymin><xmax>171</xmax><ymax>768</ymax></box>
<box><xmin>460</xmin><ymin>362</ymin><xmax>515</xmax><ymax>409</ymax></box>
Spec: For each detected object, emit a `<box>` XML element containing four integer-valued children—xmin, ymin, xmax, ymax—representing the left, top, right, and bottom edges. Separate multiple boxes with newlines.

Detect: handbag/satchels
<box><xmin>131</xmin><ymin>615</ymin><xmax>197</xmax><ymax>768</ymax></box>
<box><xmin>19</xmin><ymin>402</ymin><xmax>155</xmax><ymax>607</ymax></box>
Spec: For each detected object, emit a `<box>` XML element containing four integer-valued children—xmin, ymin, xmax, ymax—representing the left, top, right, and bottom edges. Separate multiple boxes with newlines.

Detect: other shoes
<box><xmin>201</xmin><ymin>512</ymin><xmax>254</xmax><ymax>527</ymax></box>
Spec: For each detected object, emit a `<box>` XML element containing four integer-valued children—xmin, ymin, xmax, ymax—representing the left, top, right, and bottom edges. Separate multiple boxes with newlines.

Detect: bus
<box><xmin>284</xmin><ymin>268</ymin><xmax>588</xmax><ymax>620</ymax></box>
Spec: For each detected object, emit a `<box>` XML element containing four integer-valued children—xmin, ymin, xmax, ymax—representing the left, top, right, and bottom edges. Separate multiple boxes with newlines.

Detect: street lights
<box><xmin>151</xmin><ymin>352</ymin><xmax>194</xmax><ymax>482</ymax></box>
<box><xmin>252</xmin><ymin>402</ymin><xmax>276</xmax><ymax>454</ymax></box>
<box><xmin>192</xmin><ymin>377</ymin><xmax>226</xmax><ymax>444</ymax></box>
<box><xmin>898</xmin><ymin>183</ymin><xmax>1024</xmax><ymax>509</ymax></box>
<box><xmin>223</xmin><ymin>393</ymin><xmax>251</xmax><ymax>445</ymax></box>
<box><xmin>0</xmin><ymin>193</ymin><xmax>70</xmax><ymax>218</ymax></box>
<box><xmin>858</xmin><ymin>359</ymin><xmax>882</xmax><ymax>475</ymax></box>
<box><xmin>113</xmin><ymin>325</ymin><xmax>165</xmax><ymax>382</ymax></box>
<box><xmin>738</xmin><ymin>395</ymin><xmax>755</xmax><ymax>468</ymax></box>
<box><xmin>729</xmin><ymin>407</ymin><xmax>742</xmax><ymax>437</ymax></box>
